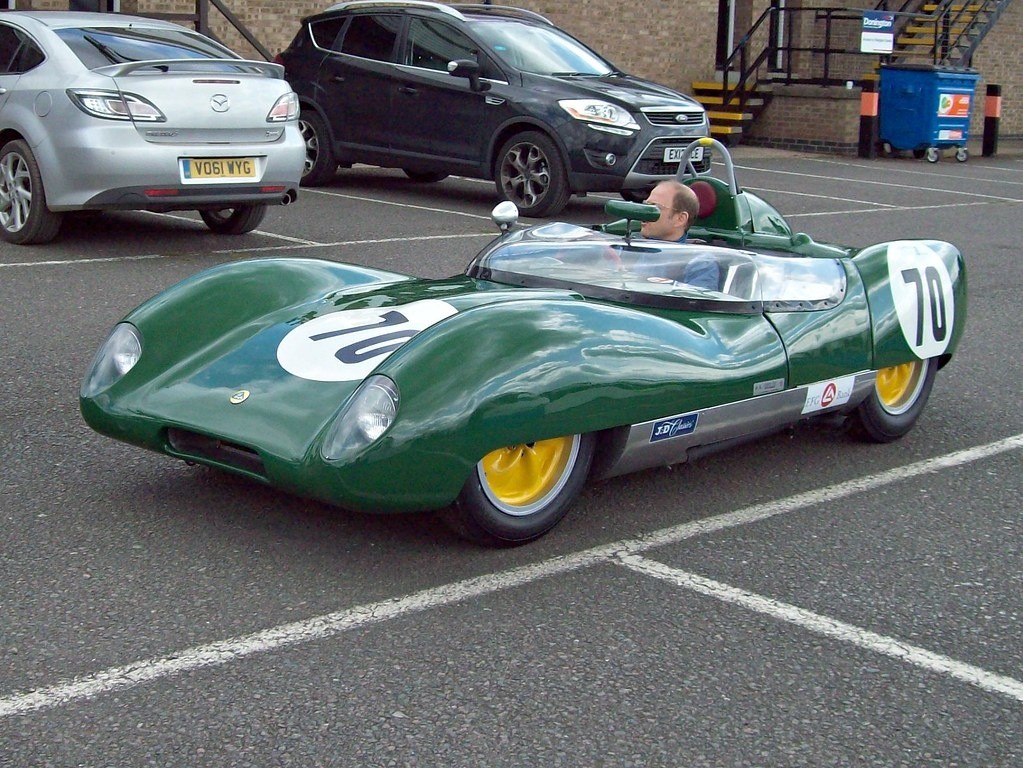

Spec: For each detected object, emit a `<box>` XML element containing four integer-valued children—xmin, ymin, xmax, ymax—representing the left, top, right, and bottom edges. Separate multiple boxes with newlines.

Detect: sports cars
<box><xmin>78</xmin><ymin>138</ymin><xmax>969</xmax><ymax>549</ymax></box>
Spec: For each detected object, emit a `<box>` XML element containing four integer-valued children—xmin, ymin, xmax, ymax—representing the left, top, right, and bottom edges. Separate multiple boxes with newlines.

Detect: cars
<box><xmin>0</xmin><ymin>7</ymin><xmax>307</xmax><ymax>247</ymax></box>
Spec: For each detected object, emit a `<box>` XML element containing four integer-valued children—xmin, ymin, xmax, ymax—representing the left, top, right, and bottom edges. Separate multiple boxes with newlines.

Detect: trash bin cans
<box><xmin>877</xmin><ymin>61</ymin><xmax>981</xmax><ymax>165</ymax></box>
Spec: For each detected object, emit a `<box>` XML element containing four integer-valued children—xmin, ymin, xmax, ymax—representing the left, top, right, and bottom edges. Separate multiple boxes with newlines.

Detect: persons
<box><xmin>637</xmin><ymin>180</ymin><xmax>701</xmax><ymax>245</ymax></box>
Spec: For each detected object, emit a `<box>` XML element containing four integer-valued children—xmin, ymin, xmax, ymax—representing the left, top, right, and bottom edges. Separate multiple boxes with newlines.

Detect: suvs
<box><xmin>275</xmin><ymin>0</ymin><xmax>713</xmax><ymax>218</ymax></box>
